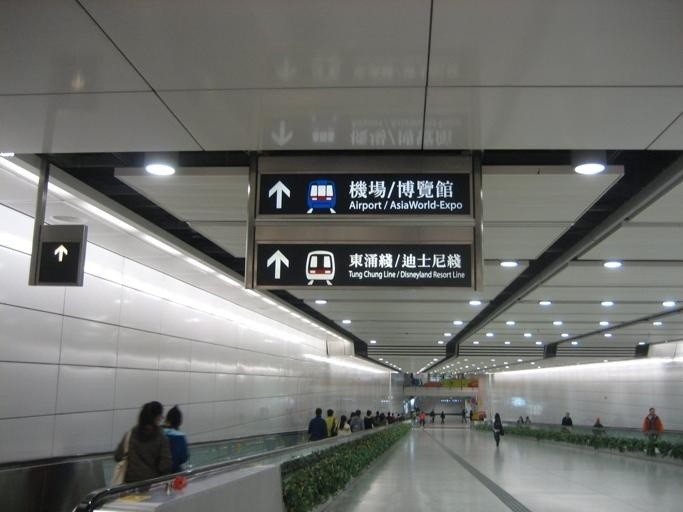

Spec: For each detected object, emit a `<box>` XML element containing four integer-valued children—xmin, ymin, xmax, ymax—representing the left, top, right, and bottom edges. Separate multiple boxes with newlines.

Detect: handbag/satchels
<box><xmin>331</xmin><ymin>418</ymin><xmax>338</xmax><ymax>436</ymax></box>
<box><xmin>500</xmin><ymin>426</ymin><xmax>503</xmax><ymax>436</ymax></box>
<box><xmin>111</xmin><ymin>430</ymin><xmax>131</xmax><ymax>487</ymax></box>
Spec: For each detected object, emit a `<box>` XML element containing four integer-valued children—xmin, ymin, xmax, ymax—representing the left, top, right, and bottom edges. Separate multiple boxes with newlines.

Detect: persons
<box><xmin>412</xmin><ymin>407</ymin><xmax>425</xmax><ymax>427</ymax></box>
<box><xmin>643</xmin><ymin>406</ymin><xmax>663</xmax><ymax>457</ymax></box>
<box><xmin>440</xmin><ymin>411</ymin><xmax>445</xmax><ymax>423</ymax></box>
<box><xmin>429</xmin><ymin>409</ymin><xmax>435</xmax><ymax>423</ymax></box>
<box><xmin>159</xmin><ymin>407</ymin><xmax>190</xmax><ymax>475</ymax></box>
<box><xmin>114</xmin><ymin>401</ymin><xmax>172</xmax><ymax>492</ymax></box>
<box><xmin>492</xmin><ymin>413</ymin><xmax>501</xmax><ymax>447</ymax></box>
<box><xmin>517</xmin><ymin>416</ymin><xmax>524</xmax><ymax>424</ymax></box>
<box><xmin>592</xmin><ymin>418</ymin><xmax>606</xmax><ymax>437</ymax></box>
<box><xmin>561</xmin><ymin>412</ymin><xmax>572</xmax><ymax>427</ymax></box>
<box><xmin>525</xmin><ymin>416</ymin><xmax>531</xmax><ymax>424</ymax></box>
<box><xmin>308</xmin><ymin>404</ymin><xmax>405</xmax><ymax>444</ymax></box>
<box><xmin>461</xmin><ymin>409</ymin><xmax>467</xmax><ymax>423</ymax></box>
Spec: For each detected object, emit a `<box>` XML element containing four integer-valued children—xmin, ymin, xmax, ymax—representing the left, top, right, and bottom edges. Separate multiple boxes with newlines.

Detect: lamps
<box><xmin>145</xmin><ymin>152</ymin><xmax>179</xmax><ymax>176</ymax></box>
<box><xmin>571</xmin><ymin>149</ymin><xmax>607</xmax><ymax>176</ymax></box>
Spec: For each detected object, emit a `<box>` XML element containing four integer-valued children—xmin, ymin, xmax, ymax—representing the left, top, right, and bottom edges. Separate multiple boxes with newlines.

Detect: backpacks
<box><xmin>494</xmin><ymin>422</ymin><xmax>501</xmax><ymax>429</ymax></box>
